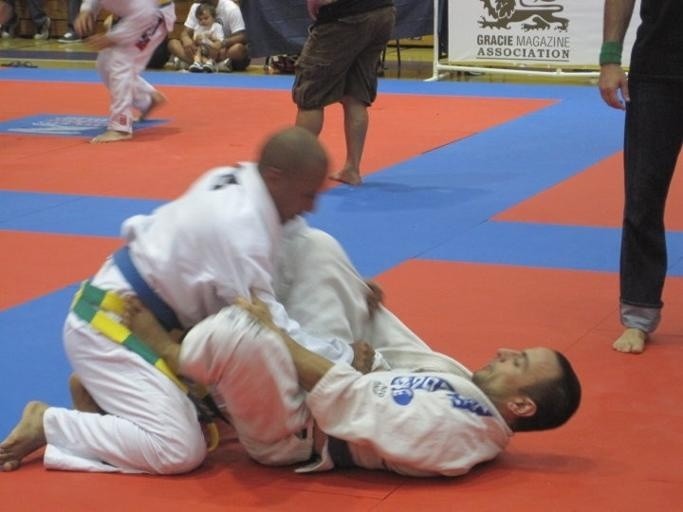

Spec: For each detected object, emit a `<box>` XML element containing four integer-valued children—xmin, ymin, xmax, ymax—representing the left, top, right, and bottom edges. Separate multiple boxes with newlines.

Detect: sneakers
<box><xmin>164</xmin><ymin>57</ymin><xmax>232</xmax><ymax>72</ymax></box>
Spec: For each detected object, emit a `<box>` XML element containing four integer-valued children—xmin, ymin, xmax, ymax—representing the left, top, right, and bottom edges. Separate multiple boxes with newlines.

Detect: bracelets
<box><xmin>600</xmin><ymin>41</ymin><xmax>624</xmax><ymax>64</ymax></box>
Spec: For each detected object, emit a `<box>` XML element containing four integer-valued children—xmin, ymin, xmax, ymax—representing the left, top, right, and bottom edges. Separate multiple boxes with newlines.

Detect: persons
<box><xmin>600</xmin><ymin>1</ymin><xmax>683</xmax><ymax>352</ymax></box>
<box><xmin>189</xmin><ymin>5</ymin><xmax>224</xmax><ymax>71</ymax></box>
<box><xmin>290</xmin><ymin>1</ymin><xmax>398</xmax><ymax>185</ymax></box>
<box><xmin>0</xmin><ymin>1</ymin><xmax>51</xmax><ymax>40</ymax></box>
<box><xmin>0</xmin><ymin>124</ymin><xmax>389</xmax><ymax>474</ymax></box>
<box><xmin>121</xmin><ymin>225</ymin><xmax>577</xmax><ymax>479</ymax></box>
<box><xmin>71</xmin><ymin>1</ymin><xmax>178</xmax><ymax>144</ymax></box>
<box><xmin>167</xmin><ymin>1</ymin><xmax>250</xmax><ymax>72</ymax></box>
